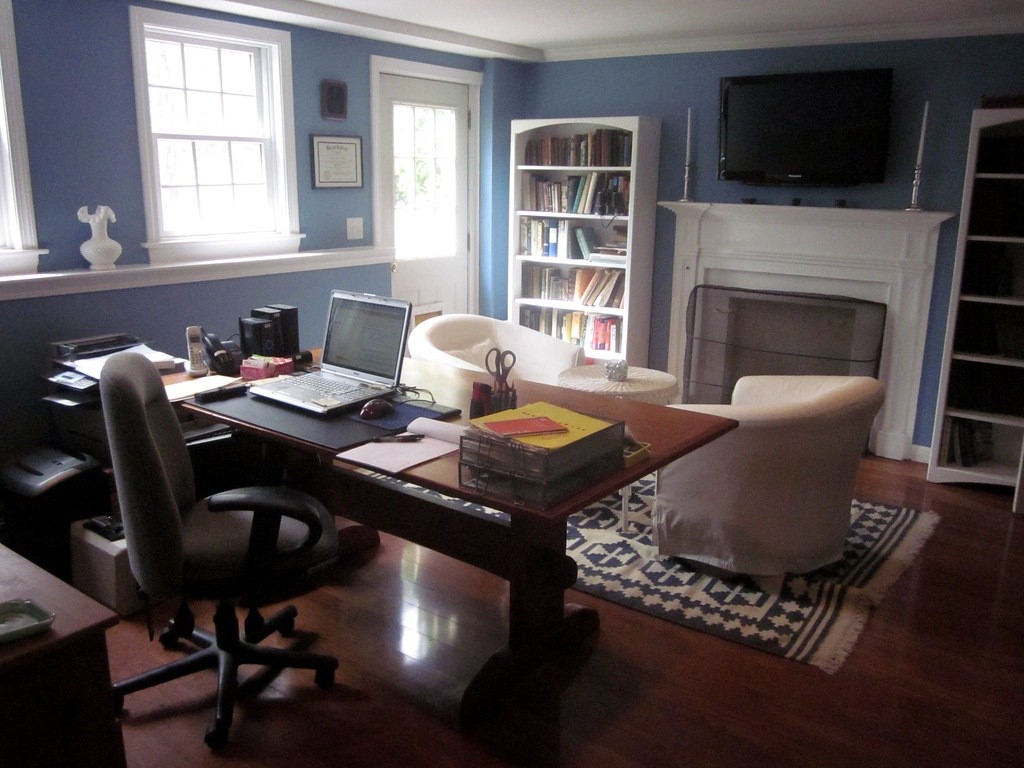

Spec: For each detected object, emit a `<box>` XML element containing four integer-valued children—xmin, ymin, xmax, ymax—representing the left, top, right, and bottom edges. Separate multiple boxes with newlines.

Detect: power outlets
<box><xmin>346</xmin><ymin>218</ymin><xmax>363</xmax><ymax>240</ymax></box>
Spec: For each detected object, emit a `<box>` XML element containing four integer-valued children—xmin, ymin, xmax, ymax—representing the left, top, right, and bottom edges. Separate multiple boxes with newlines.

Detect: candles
<box><xmin>685</xmin><ymin>107</ymin><xmax>692</xmax><ymax>167</ymax></box>
<box><xmin>917</xmin><ymin>100</ymin><xmax>929</xmax><ymax>166</ymax></box>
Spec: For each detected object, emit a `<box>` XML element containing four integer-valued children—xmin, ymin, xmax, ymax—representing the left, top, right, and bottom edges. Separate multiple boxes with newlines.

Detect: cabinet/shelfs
<box><xmin>925</xmin><ymin>107</ymin><xmax>1024</xmax><ymax>491</ymax></box>
<box><xmin>506</xmin><ymin>114</ymin><xmax>664</xmax><ymax>370</ymax></box>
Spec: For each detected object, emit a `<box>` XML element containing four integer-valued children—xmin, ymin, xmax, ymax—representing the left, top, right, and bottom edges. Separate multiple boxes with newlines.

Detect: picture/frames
<box><xmin>309</xmin><ymin>133</ymin><xmax>364</xmax><ymax>190</ymax></box>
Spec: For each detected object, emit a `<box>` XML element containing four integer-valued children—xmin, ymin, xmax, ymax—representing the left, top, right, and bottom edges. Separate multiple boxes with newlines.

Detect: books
<box><xmin>524</xmin><ymin>127</ymin><xmax>631</xmax><ymax>165</ymax></box>
<box><xmin>522</xmin><ymin>172</ymin><xmax>630</xmax><ymax>215</ymax></box>
<box><xmin>522</xmin><ymin>261</ymin><xmax>625</xmax><ymax>308</ymax></box>
<box><xmin>941</xmin><ymin>418</ymin><xmax>993</xmax><ymax>467</ymax></box>
<box><xmin>522</xmin><ymin>217</ymin><xmax>625</xmax><ymax>263</ymax></box>
<box><xmin>519</xmin><ymin>307</ymin><xmax>622</xmax><ymax>353</ymax></box>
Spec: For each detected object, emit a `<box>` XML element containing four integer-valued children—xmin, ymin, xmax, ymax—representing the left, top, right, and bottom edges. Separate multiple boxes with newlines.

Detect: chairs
<box><xmin>98</xmin><ymin>349</ymin><xmax>383</xmax><ymax>749</ymax></box>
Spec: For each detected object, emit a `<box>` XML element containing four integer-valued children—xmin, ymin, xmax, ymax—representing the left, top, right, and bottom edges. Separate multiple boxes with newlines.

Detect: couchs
<box><xmin>654</xmin><ymin>374</ymin><xmax>892</xmax><ymax>570</ymax></box>
<box><xmin>407</xmin><ymin>311</ymin><xmax>586</xmax><ymax>388</ymax></box>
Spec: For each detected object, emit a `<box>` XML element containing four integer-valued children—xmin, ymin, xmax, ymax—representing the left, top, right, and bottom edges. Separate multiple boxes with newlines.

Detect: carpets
<box><xmin>354</xmin><ymin>437</ymin><xmax>942</xmax><ymax>677</ymax></box>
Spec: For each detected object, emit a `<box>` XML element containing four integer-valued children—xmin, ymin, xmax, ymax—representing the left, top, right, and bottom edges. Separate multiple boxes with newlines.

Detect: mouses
<box><xmin>360</xmin><ymin>398</ymin><xmax>395</xmax><ymax>420</ymax></box>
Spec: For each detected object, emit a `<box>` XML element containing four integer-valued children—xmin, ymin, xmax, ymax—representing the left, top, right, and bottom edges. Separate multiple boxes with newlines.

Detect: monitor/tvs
<box><xmin>717</xmin><ymin>68</ymin><xmax>895</xmax><ymax>188</ymax></box>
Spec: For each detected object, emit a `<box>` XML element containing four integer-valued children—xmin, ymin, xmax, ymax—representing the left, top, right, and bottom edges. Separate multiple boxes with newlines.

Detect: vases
<box><xmin>77</xmin><ymin>204</ymin><xmax>122</xmax><ymax>272</ymax></box>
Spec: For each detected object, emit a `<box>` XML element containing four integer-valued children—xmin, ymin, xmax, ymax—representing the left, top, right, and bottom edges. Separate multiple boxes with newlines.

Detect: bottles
<box><xmin>77</xmin><ymin>205</ymin><xmax>122</xmax><ymax>270</ymax></box>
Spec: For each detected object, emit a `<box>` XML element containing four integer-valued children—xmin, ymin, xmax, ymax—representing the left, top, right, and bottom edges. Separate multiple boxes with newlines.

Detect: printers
<box><xmin>43</xmin><ymin>331</ymin><xmax>142</xmax><ymax>397</ymax></box>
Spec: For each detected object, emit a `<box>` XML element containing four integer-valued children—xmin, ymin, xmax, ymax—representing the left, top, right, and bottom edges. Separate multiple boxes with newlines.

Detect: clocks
<box><xmin>322</xmin><ymin>78</ymin><xmax>348</xmax><ymax>118</ymax></box>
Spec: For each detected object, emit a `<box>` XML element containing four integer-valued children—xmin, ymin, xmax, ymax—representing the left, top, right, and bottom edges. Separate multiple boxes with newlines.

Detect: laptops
<box><xmin>250</xmin><ymin>289</ymin><xmax>412</xmax><ymax>416</ymax></box>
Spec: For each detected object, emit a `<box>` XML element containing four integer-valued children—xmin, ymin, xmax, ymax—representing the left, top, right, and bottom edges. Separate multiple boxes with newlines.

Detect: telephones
<box><xmin>200</xmin><ymin>327</ymin><xmax>244</xmax><ymax>377</ymax></box>
<box><xmin>184</xmin><ymin>326</ymin><xmax>208</xmax><ymax>376</ymax></box>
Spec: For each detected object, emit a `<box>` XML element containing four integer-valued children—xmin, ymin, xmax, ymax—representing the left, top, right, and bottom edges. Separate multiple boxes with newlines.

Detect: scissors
<box><xmin>484</xmin><ymin>347</ymin><xmax>516</xmax><ymax>390</ymax></box>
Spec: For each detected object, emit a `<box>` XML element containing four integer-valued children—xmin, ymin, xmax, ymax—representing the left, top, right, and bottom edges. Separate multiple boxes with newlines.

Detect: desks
<box><xmin>160</xmin><ymin>347</ymin><xmax>738</xmax><ymax>725</ymax></box>
<box><xmin>559</xmin><ymin>363</ymin><xmax>677</xmax><ymax>532</ymax></box>
<box><xmin>0</xmin><ymin>541</ymin><xmax>128</xmax><ymax>768</ymax></box>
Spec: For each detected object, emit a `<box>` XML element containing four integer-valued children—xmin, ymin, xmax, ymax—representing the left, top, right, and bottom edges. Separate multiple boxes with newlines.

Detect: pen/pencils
<box><xmin>488</xmin><ymin>381</ymin><xmax>517</xmax><ymax>411</ymax></box>
<box><xmin>373</xmin><ymin>434</ymin><xmax>425</xmax><ymax>442</ymax></box>
